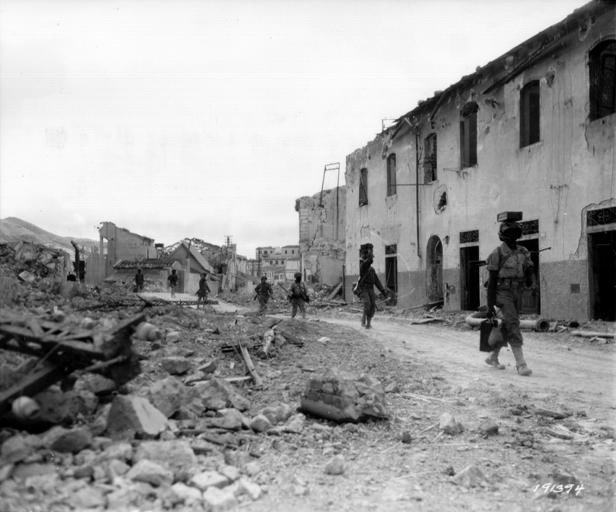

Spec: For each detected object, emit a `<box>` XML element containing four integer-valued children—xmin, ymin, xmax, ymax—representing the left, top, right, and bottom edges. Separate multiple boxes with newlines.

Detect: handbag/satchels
<box><xmin>353</xmin><ymin>276</ymin><xmax>362</xmax><ymax>295</ymax></box>
<box><xmin>480</xmin><ymin>320</ymin><xmax>498</xmax><ymax>352</ymax></box>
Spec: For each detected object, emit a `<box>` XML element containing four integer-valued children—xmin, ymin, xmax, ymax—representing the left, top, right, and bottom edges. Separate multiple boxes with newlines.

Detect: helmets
<box><xmin>499</xmin><ymin>222</ymin><xmax>522</xmax><ymax>241</ymax></box>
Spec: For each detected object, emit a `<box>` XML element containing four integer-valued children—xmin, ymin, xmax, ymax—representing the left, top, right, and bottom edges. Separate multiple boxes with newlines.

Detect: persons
<box><xmin>167</xmin><ymin>269</ymin><xmax>177</xmax><ymax>297</ymax></box>
<box><xmin>354</xmin><ymin>254</ymin><xmax>388</xmax><ymax>329</ymax></box>
<box><xmin>287</xmin><ymin>272</ymin><xmax>309</xmax><ymax>319</ymax></box>
<box><xmin>484</xmin><ymin>223</ymin><xmax>537</xmax><ymax>377</ymax></box>
<box><xmin>194</xmin><ymin>272</ymin><xmax>211</xmax><ymax>310</ymax></box>
<box><xmin>134</xmin><ymin>269</ymin><xmax>144</xmax><ymax>292</ymax></box>
<box><xmin>254</xmin><ymin>276</ymin><xmax>273</xmax><ymax>316</ymax></box>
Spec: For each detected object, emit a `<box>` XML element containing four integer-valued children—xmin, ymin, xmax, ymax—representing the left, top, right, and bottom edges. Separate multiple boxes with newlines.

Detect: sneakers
<box><xmin>515</xmin><ymin>364</ymin><xmax>531</xmax><ymax>375</ymax></box>
<box><xmin>486</xmin><ymin>359</ymin><xmax>505</xmax><ymax>369</ymax></box>
